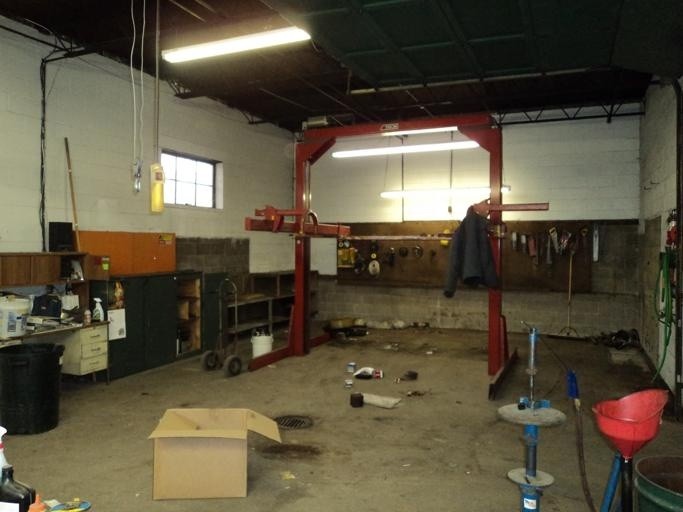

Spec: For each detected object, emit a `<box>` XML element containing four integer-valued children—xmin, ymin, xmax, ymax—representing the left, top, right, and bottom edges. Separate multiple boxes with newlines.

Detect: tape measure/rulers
<box><xmin>372</xmin><ymin>254</ymin><xmax>378</xmax><ymax>260</ymax></box>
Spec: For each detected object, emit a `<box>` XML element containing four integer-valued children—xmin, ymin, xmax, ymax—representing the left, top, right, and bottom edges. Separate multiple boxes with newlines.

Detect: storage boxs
<box><xmin>148</xmin><ymin>408</ymin><xmax>283</xmax><ymax>500</ymax></box>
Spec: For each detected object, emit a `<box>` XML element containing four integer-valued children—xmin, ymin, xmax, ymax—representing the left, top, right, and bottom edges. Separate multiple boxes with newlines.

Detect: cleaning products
<box><xmin>91</xmin><ymin>296</ymin><xmax>105</xmax><ymax>323</ymax></box>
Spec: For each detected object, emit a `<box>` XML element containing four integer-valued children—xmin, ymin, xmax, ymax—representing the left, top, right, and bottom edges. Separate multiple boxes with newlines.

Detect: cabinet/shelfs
<box><xmin>72</xmin><ymin>231</ymin><xmax>177</xmax><ymax>278</ymax></box>
<box><xmin>1</xmin><ymin>252</ymin><xmax>110</xmax><ymax>288</ymax></box>
<box><xmin>62</xmin><ymin>269</ymin><xmax>229</xmax><ymax>385</ymax></box>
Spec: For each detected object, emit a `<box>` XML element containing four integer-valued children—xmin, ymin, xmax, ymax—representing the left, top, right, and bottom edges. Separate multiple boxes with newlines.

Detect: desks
<box><xmin>1</xmin><ymin>320</ymin><xmax>110</xmax><ymax>391</ymax></box>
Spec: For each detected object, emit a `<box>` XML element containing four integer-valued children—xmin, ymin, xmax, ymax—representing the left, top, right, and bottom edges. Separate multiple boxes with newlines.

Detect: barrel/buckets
<box><xmin>0</xmin><ymin>295</ymin><xmax>33</xmax><ymax>340</ymax></box>
<box><xmin>250</xmin><ymin>335</ymin><xmax>273</xmax><ymax>359</ymax></box>
